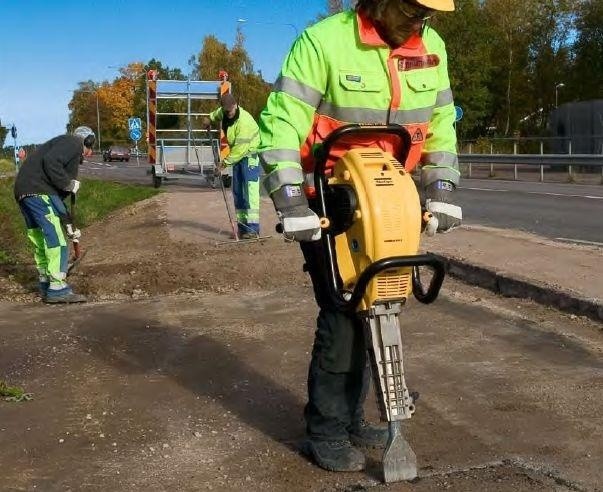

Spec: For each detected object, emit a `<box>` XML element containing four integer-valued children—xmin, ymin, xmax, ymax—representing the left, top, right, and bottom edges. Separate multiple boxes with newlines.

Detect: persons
<box><xmin>14</xmin><ymin>125</ymin><xmax>96</xmax><ymax>304</ymax></box>
<box><xmin>257</xmin><ymin>0</ymin><xmax>464</xmax><ymax>471</ymax></box>
<box><xmin>202</xmin><ymin>94</ymin><xmax>261</xmax><ymax>239</ymax></box>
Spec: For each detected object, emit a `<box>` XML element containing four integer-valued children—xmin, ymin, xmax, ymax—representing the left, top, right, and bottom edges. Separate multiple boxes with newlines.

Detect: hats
<box><xmin>221</xmin><ymin>95</ymin><xmax>237</xmax><ymax>112</ymax></box>
<box><xmin>415</xmin><ymin>0</ymin><xmax>456</xmax><ymax>13</ymax></box>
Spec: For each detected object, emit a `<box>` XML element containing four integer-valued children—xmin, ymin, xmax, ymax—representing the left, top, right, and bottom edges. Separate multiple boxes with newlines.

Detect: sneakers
<box><xmin>38</xmin><ymin>281</ymin><xmax>88</xmax><ymax>303</ymax></box>
<box><xmin>242</xmin><ymin>231</ymin><xmax>258</xmax><ymax>239</ymax></box>
<box><xmin>312</xmin><ymin>442</ymin><xmax>365</xmax><ymax>472</ymax></box>
<box><xmin>237</xmin><ymin>229</ymin><xmax>246</xmax><ymax>239</ymax></box>
<box><xmin>350</xmin><ymin>420</ymin><xmax>392</xmax><ymax>442</ymax></box>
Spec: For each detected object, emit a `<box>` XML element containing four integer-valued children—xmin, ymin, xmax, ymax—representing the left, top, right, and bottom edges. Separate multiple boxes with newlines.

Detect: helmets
<box><xmin>73</xmin><ymin>126</ymin><xmax>95</xmax><ymax>150</ymax></box>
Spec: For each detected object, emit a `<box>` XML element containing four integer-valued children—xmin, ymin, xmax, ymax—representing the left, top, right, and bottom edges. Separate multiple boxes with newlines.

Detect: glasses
<box><xmin>400</xmin><ymin>3</ymin><xmax>436</xmax><ymax>19</ymax></box>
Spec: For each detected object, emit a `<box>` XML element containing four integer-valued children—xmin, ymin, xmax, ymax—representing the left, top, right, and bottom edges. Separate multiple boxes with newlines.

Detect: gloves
<box><xmin>63</xmin><ymin>179</ymin><xmax>80</xmax><ymax>194</ymax></box>
<box><xmin>66</xmin><ymin>224</ymin><xmax>81</xmax><ymax>243</ymax></box>
<box><xmin>201</xmin><ymin>117</ymin><xmax>212</xmax><ymax>129</ymax></box>
<box><xmin>272</xmin><ymin>187</ymin><xmax>322</xmax><ymax>241</ymax></box>
<box><xmin>425</xmin><ymin>180</ymin><xmax>464</xmax><ymax>236</ymax></box>
<box><xmin>214</xmin><ymin>162</ymin><xmax>225</xmax><ymax>176</ymax></box>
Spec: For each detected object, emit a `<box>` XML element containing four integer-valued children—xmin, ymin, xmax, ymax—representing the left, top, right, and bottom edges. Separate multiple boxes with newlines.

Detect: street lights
<box><xmin>554</xmin><ymin>82</ymin><xmax>564</xmax><ymax>108</ymax></box>
<box><xmin>107</xmin><ymin>65</ymin><xmax>151</xmax><ymax>148</ymax></box>
<box><xmin>237</xmin><ymin>18</ymin><xmax>299</xmax><ymax>41</ymax></box>
<box><xmin>81</xmin><ymin>89</ymin><xmax>102</xmax><ymax>154</ymax></box>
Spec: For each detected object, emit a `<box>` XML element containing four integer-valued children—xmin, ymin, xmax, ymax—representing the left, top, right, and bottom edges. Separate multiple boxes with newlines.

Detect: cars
<box><xmin>103</xmin><ymin>146</ymin><xmax>131</xmax><ymax>163</ymax></box>
<box><xmin>78</xmin><ymin>153</ymin><xmax>83</xmax><ymax>166</ymax></box>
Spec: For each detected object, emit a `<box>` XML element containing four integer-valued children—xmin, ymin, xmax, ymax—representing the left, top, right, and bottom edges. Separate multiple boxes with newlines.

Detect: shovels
<box><xmin>67</xmin><ymin>192</ymin><xmax>88</xmax><ymax>272</ymax></box>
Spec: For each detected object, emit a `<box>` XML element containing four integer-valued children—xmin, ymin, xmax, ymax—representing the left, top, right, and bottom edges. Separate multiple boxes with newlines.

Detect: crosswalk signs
<box><xmin>128</xmin><ymin>118</ymin><xmax>140</xmax><ymax>130</ymax></box>
